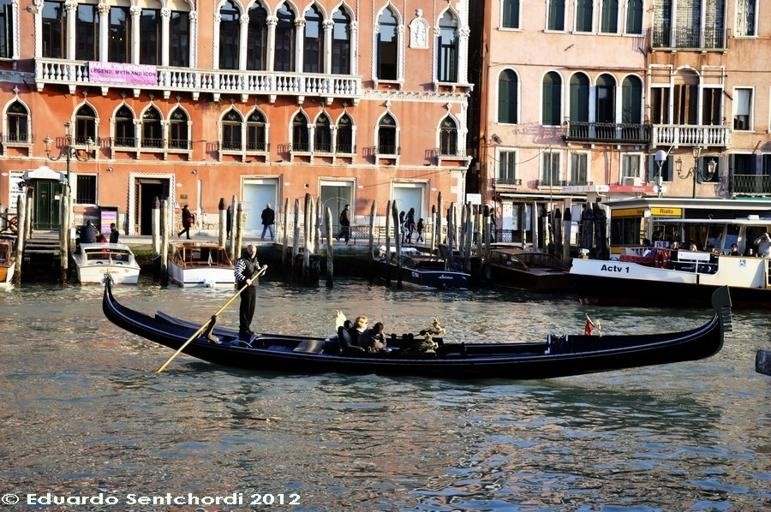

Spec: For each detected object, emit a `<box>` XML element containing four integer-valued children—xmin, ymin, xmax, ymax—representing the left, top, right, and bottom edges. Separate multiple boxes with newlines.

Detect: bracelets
<box><xmin>382</xmin><ymin>336</ymin><xmax>386</xmax><ymax>339</ymax></box>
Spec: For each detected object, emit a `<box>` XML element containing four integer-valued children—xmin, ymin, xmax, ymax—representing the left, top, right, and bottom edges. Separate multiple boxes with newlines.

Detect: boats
<box><xmin>0</xmin><ymin>229</ymin><xmax>17</xmax><ymax>286</ymax></box>
<box><xmin>167</xmin><ymin>239</ymin><xmax>238</xmax><ymax>289</ymax></box>
<box><xmin>570</xmin><ymin>215</ymin><xmax>771</xmax><ymax>300</ymax></box>
<box><xmin>101</xmin><ymin>275</ymin><xmax>732</xmax><ymax>378</ymax></box>
<box><xmin>374</xmin><ymin>244</ymin><xmax>471</xmax><ymax>291</ymax></box>
<box><xmin>482</xmin><ymin>248</ymin><xmax>571</xmax><ymax>292</ymax></box>
<box><xmin>69</xmin><ymin>224</ymin><xmax>142</xmax><ymax>286</ymax></box>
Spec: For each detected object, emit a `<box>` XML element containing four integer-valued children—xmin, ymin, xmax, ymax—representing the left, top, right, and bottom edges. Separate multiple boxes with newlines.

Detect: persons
<box><xmin>234</xmin><ymin>244</ymin><xmax>267</xmax><ymax>340</ymax></box>
<box><xmin>177</xmin><ymin>205</ymin><xmax>197</xmax><ymax>239</ymax></box>
<box><xmin>360</xmin><ymin>321</ymin><xmax>388</xmax><ymax>352</ymax></box>
<box><xmin>81</xmin><ymin>219</ymin><xmax>99</xmax><ymax>253</ymax></box>
<box><xmin>261</xmin><ymin>202</ymin><xmax>276</xmax><ymax>241</ymax></box>
<box><xmin>416</xmin><ymin>217</ymin><xmax>426</xmax><ymax>242</ymax></box>
<box><xmin>405</xmin><ymin>208</ymin><xmax>418</xmax><ymax>244</ymax></box>
<box><xmin>98</xmin><ymin>233</ymin><xmax>109</xmax><ymax>256</ymax></box>
<box><xmin>641</xmin><ymin>233</ymin><xmax>771</xmax><ymax>274</ymax></box>
<box><xmin>353</xmin><ymin>317</ymin><xmax>369</xmax><ymax>339</ymax></box>
<box><xmin>335</xmin><ymin>203</ymin><xmax>354</xmax><ymax>245</ymax></box>
<box><xmin>400</xmin><ymin>211</ymin><xmax>409</xmax><ymax>244</ymax></box>
<box><xmin>110</xmin><ymin>223</ymin><xmax>120</xmax><ymax>252</ymax></box>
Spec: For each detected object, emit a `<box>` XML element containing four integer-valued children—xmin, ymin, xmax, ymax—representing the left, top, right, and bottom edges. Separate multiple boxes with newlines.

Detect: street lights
<box><xmin>655</xmin><ymin>150</ymin><xmax>668</xmax><ymax>198</ymax></box>
<box><xmin>43</xmin><ymin>119</ymin><xmax>93</xmax><ymax>234</ymax></box>
<box><xmin>676</xmin><ymin>144</ymin><xmax>718</xmax><ymax>199</ymax></box>
